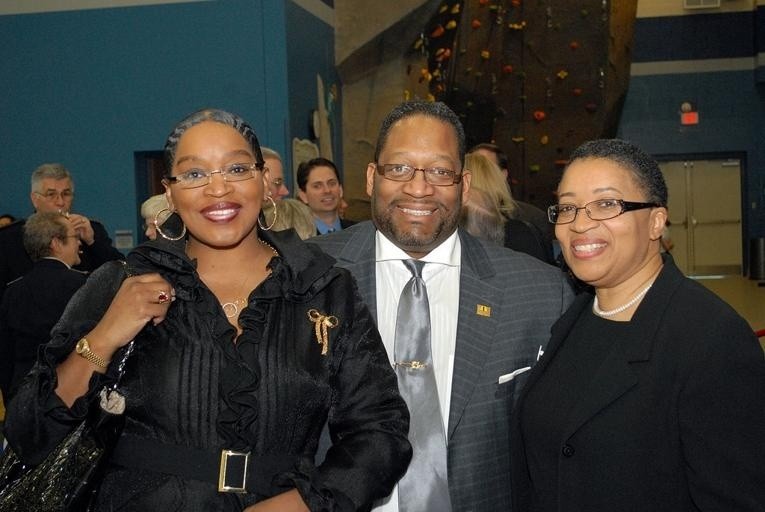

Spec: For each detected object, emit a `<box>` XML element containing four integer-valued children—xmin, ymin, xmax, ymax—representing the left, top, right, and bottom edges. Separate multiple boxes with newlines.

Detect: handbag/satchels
<box><xmin>0</xmin><ymin>371</ymin><xmax>127</xmax><ymax>512</ymax></box>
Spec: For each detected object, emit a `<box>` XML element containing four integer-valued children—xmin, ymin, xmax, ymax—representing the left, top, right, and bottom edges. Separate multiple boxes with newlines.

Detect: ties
<box><xmin>393</xmin><ymin>259</ymin><xmax>452</xmax><ymax>512</ymax></box>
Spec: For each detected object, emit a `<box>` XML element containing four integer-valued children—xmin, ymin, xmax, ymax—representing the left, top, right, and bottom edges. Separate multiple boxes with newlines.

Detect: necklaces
<box><xmin>593</xmin><ymin>284</ymin><xmax>651</xmax><ymax>318</ymax></box>
<box><xmin>185</xmin><ymin>241</ymin><xmax>279</xmax><ymax>318</ymax></box>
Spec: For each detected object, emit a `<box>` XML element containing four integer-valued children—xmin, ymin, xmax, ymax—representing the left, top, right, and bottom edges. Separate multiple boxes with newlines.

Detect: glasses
<box><xmin>167</xmin><ymin>163</ymin><xmax>261</xmax><ymax>189</ymax></box>
<box><xmin>548</xmin><ymin>198</ymin><xmax>657</xmax><ymax>224</ymax></box>
<box><xmin>35</xmin><ymin>191</ymin><xmax>73</xmax><ymax>202</ymax></box>
<box><xmin>377</xmin><ymin>163</ymin><xmax>461</xmax><ymax>186</ymax></box>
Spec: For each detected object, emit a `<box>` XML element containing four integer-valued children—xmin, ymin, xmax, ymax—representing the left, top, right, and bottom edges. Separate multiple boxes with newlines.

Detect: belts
<box><xmin>113</xmin><ymin>435</ymin><xmax>295</xmax><ymax>496</ymax></box>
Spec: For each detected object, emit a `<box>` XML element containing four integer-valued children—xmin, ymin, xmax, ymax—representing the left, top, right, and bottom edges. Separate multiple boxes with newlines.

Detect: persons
<box><xmin>302</xmin><ymin>101</ymin><xmax>578</xmax><ymax>512</ymax></box>
<box><xmin>1</xmin><ymin>108</ymin><xmax>413</xmax><ymax>512</ymax></box>
<box><xmin>512</xmin><ymin>139</ymin><xmax>765</xmax><ymax>512</ymax></box>
<box><xmin>1</xmin><ymin>165</ymin><xmax>127</xmax><ymax>419</ymax></box>
<box><xmin>463</xmin><ymin>153</ymin><xmax>548</xmax><ymax>263</ymax></box>
<box><xmin>141</xmin><ymin>193</ymin><xmax>172</xmax><ymax>242</ymax></box>
<box><xmin>468</xmin><ymin>143</ymin><xmax>555</xmax><ymax>264</ymax></box>
<box><xmin>257</xmin><ymin>148</ymin><xmax>357</xmax><ymax>239</ymax></box>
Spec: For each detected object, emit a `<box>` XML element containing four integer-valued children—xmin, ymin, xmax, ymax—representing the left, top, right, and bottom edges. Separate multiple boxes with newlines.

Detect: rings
<box><xmin>159</xmin><ymin>292</ymin><xmax>169</xmax><ymax>303</ymax></box>
